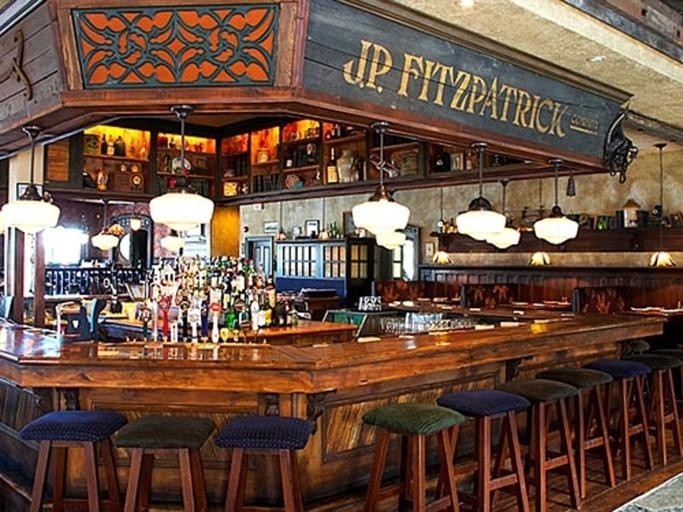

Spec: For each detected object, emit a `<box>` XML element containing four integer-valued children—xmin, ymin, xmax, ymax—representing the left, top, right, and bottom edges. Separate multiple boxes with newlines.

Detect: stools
<box><xmin>216</xmin><ymin>416</ymin><xmax>313</xmax><ymax>512</ymax></box>
<box><xmin>535</xmin><ymin>367</ymin><xmax>617</xmax><ymax>499</ymax></box>
<box><xmin>436</xmin><ymin>389</ymin><xmax>531</xmax><ymax>511</ymax></box>
<box><xmin>629</xmin><ymin>352</ymin><xmax>683</xmax><ymax>467</ymax></box>
<box><xmin>20</xmin><ymin>411</ymin><xmax>126</xmax><ymax>512</ymax></box>
<box><xmin>586</xmin><ymin>358</ymin><xmax>655</xmax><ymax>481</ymax></box>
<box><xmin>490</xmin><ymin>381</ymin><xmax>581</xmax><ymax>512</ymax></box>
<box><xmin>363</xmin><ymin>403</ymin><xmax>465</xmax><ymax>512</ymax></box>
<box><xmin>110</xmin><ymin>414</ymin><xmax>216</xmax><ymax>512</ymax></box>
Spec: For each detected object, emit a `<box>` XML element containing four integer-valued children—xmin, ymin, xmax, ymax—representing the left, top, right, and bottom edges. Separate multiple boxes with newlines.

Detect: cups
<box><xmin>406</xmin><ymin>312</ymin><xmax>442</xmax><ymax>334</ymax></box>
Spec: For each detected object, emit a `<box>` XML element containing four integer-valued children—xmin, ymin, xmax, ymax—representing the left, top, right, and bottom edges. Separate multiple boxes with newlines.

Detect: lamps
<box><xmin>650</xmin><ymin>141</ymin><xmax>678</xmax><ymax>270</ymax></box>
<box><xmin>534</xmin><ymin>159</ymin><xmax>579</xmax><ymax>245</ymax></box>
<box><xmin>276</xmin><ymin>203</ymin><xmax>288</xmax><ymax>241</ymax></box>
<box><xmin>1</xmin><ymin>125</ymin><xmax>61</xmax><ymax>235</ymax></box>
<box><xmin>148</xmin><ymin>106</ymin><xmax>215</xmax><ymax>231</ymax></box>
<box><xmin>456</xmin><ymin>143</ymin><xmax>505</xmax><ymax>243</ymax></box>
<box><xmin>433</xmin><ymin>187</ymin><xmax>453</xmax><ymax>266</ymax></box>
<box><xmin>486</xmin><ymin>179</ymin><xmax>520</xmax><ymax>250</ymax></box>
<box><xmin>319</xmin><ymin>198</ymin><xmax>330</xmax><ymax>243</ymax></box>
<box><xmin>529</xmin><ymin>166</ymin><xmax>551</xmax><ymax>266</ymax></box>
<box><xmin>160</xmin><ymin>229</ymin><xmax>184</xmax><ymax>249</ymax></box>
<box><xmin>352</xmin><ymin>122</ymin><xmax>412</xmax><ymax>235</ymax></box>
<box><xmin>375</xmin><ymin>178</ymin><xmax>406</xmax><ymax>250</ymax></box>
<box><xmin>90</xmin><ymin>199</ymin><xmax>120</xmax><ymax>252</ymax></box>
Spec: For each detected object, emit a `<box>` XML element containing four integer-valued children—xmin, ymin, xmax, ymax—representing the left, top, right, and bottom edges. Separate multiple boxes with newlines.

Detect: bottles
<box><xmin>115</xmin><ymin>136</ymin><xmax>125</xmax><ymax>157</ymax></box>
<box><xmin>327</xmin><ymin>221</ymin><xmax>339</xmax><ymax>239</ymax></box>
<box><xmin>101</xmin><ymin>134</ymin><xmax>107</xmax><ymax>155</ymax></box>
<box><xmin>110</xmin><ymin>289</ymin><xmax>123</xmax><ymax>314</ymax></box>
<box><xmin>326</xmin><ymin>147</ymin><xmax>339</xmax><ymax>183</ymax></box>
<box><xmin>136</xmin><ymin>255</ymin><xmax>301</xmax><ymax>331</ymax></box>
<box><xmin>97</xmin><ymin>166</ymin><xmax>107</xmax><ymax>191</ymax></box>
<box><xmin>107</xmin><ymin>135</ymin><xmax>115</xmax><ymax>155</ymax></box>
